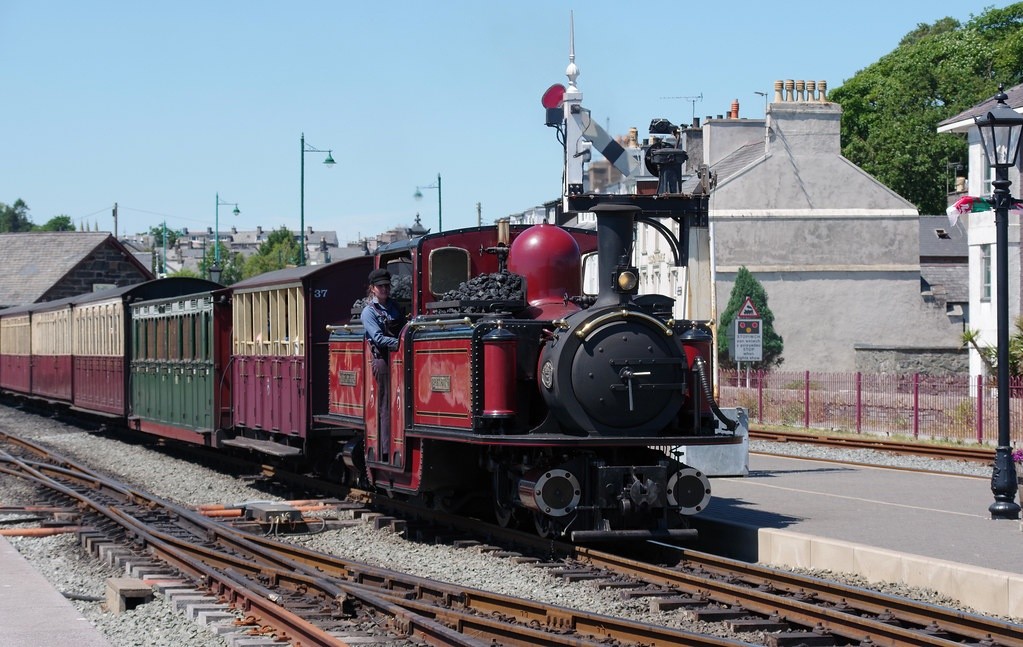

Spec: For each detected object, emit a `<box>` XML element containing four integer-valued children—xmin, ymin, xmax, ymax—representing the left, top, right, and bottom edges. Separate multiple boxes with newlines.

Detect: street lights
<box><xmin>412</xmin><ymin>171</ymin><xmax>443</xmax><ymax>234</ymax></box>
<box><xmin>298</xmin><ymin>130</ymin><xmax>339</xmax><ymax>267</ymax></box>
<box><xmin>973</xmin><ymin>82</ymin><xmax>1023</xmax><ymax>521</ymax></box>
<box><xmin>162</xmin><ymin>220</ymin><xmax>189</xmax><ymax>279</ymax></box>
<box><xmin>213</xmin><ymin>192</ymin><xmax>241</xmax><ymax>266</ymax></box>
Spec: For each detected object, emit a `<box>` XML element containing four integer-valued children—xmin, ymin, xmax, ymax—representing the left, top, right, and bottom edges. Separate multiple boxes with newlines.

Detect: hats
<box><xmin>368</xmin><ymin>269</ymin><xmax>390</xmax><ymax>285</ymax></box>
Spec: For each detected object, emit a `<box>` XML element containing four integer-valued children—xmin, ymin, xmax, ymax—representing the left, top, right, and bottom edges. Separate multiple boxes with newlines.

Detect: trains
<box><xmin>1</xmin><ymin>137</ymin><xmax>750</xmax><ymax>556</ymax></box>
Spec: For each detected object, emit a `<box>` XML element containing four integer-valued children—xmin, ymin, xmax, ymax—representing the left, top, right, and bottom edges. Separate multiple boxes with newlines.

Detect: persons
<box><xmin>360</xmin><ymin>269</ymin><xmax>407</xmax><ymax>461</ymax></box>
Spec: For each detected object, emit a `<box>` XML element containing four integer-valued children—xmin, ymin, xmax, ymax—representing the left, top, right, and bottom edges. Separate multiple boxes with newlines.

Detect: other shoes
<box><xmin>383</xmin><ymin>453</ymin><xmax>388</xmax><ymax>462</ymax></box>
